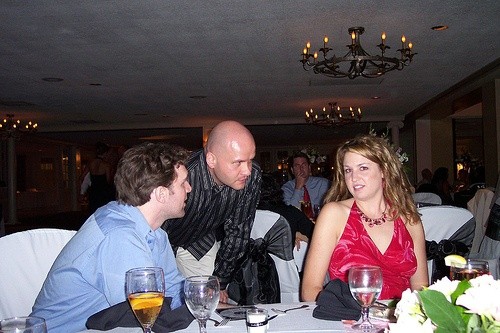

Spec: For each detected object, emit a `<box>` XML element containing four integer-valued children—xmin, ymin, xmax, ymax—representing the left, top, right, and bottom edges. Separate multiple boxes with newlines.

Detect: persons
<box><xmin>300</xmin><ymin>134</ymin><xmax>429</xmax><ymax>302</ymax></box>
<box><xmin>280</xmin><ymin>152</ymin><xmax>334</xmax><ymax>224</ymax></box>
<box><xmin>18</xmin><ymin>120</ymin><xmax>262</xmax><ymax>333</ymax></box>
<box><xmin>417</xmin><ymin>166</ymin><xmax>454</xmax><ymax>205</ymax></box>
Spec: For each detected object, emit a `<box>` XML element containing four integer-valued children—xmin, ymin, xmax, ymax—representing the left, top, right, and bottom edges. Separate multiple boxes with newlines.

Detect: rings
<box><xmin>301</xmin><ymin>173</ymin><xmax>303</xmax><ymax>176</ymax></box>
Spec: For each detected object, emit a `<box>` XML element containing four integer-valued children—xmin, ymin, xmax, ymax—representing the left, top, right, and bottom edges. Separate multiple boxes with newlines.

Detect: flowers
<box><xmin>393</xmin><ymin>274</ymin><xmax>500</xmax><ymax>333</ymax></box>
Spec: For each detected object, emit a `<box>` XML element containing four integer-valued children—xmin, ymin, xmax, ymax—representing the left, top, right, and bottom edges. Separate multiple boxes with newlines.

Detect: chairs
<box><xmin>0</xmin><ymin>228</ymin><xmax>78</xmax><ymax>322</ymax></box>
<box><xmin>412</xmin><ymin>188</ymin><xmax>500</xmax><ymax>283</ymax></box>
<box><xmin>176</xmin><ymin>210</ymin><xmax>306</xmax><ymax>302</ymax></box>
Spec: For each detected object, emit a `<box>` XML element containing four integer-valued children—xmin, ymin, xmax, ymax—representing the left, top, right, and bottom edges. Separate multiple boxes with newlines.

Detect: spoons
<box><xmin>271</xmin><ymin>305</ymin><xmax>309</xmax><ymax>313</ymax></box>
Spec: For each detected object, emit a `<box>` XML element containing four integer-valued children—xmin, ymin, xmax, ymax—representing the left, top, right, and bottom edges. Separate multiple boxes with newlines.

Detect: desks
<box><xmin>86</xmin><ymin>299</ymin><xmax>398</xmax><ymax>333</ymax></box>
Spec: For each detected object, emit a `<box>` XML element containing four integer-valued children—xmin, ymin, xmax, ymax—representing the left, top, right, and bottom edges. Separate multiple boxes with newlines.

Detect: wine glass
<box><xmin>183</xmin><ymin>275</ymin><xmax>220</xmax><ymax>333</ymax></box>
<box><xmin>125</xmin><ymin>267</ymin><xmax>166</xmax><ymax>333</ymax></box>
<box><xmin>348</xmin><ymin>266</ymin><xmax>384</xmax><ymax>333</ymax></box>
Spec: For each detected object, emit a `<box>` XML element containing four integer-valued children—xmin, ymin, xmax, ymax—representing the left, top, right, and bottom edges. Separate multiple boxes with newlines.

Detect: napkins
<box><xmin>310</xmin><ymin>279</ymin><xmax>362</xmax><ymax>322</ymax></box>
<box><xmin>86</xmin><ymin>297</ymin><xmax>196</xmax><ymax>332</ymax></box>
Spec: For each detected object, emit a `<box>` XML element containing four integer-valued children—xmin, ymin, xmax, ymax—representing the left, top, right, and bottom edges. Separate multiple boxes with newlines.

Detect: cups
<box><xmin>0</xmin><ymin>316</ymin><xmax>47</xmax><ymax>333</ymax></box>
<box><xmin>314</xmin><ymin>204</ymin><xmax>321</xmax><ymax>216</ymax></box>
<box><xmin>246</xmin><ymin>309</ymin><xmax>269</xmax><ymax>333</ymax></box>
<box><xmin>451</xmin><ymin>259</ymin><xmax>490</xmax><ymax>282</ymax></box>
<box><xmin>302</xmin><ymin>201</ymin><xmax>311</xmax><ymax>218</ymax></box>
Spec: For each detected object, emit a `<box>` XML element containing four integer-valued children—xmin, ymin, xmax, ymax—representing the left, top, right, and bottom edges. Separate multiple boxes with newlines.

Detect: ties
<box><xmin>303</xmin><ymin>184</ymin><xmax>313</xmax><ymax>219</ymax></box>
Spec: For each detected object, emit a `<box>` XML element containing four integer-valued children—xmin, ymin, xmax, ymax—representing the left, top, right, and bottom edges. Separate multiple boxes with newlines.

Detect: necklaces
<box><xmin>356</xmin><ymin>204</ymin><xmax>390</xmax><ymax>228</ymax></box>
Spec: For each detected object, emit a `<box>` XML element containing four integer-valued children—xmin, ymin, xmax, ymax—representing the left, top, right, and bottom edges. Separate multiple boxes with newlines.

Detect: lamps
<box><xmin>302</xmin><ymin>26</ymin><xmax>414</xmax><ymax>79</ymax></box>
<box><xmin>305</xmin><ymin>101</ymin><xmax>371</xmax><ymax>127</ymax></box>
<box><xmin>0</xmin><ymin>114</ymin><xmax>41</xmax><ymax>137</ymax></box>
